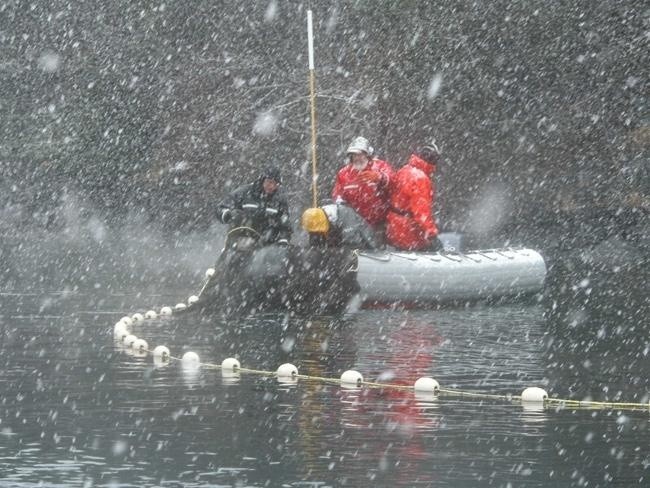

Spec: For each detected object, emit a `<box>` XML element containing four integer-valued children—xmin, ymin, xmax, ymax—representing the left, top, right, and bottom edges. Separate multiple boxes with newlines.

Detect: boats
<box><xmin>214</xmin><ymin>244</ymin><xmax>548</xmax><ymax>300</ymax></box>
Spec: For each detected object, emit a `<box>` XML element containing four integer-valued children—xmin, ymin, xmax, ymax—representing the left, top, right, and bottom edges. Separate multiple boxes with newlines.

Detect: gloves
<box><xmin>360</xmin><ymin>171</ymin><xmax>381</xmax><ymax>183</ymax></box>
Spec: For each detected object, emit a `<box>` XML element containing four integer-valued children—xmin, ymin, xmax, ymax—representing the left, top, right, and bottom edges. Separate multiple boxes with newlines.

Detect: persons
<box><xmin>332</xmin><ymin>136</ymin><xmax>392</xmax><ymax>251</ymax></box>
<box><xmin>213</xmin><ymin>165</ymin><xmax>293</xmax><ymax>251</ymax></box>
<box><xmin>383</xmin><ymin>146</ymin><xmax>447</xmax><ymax>254</ymax></box>
<box><xmin>300</xmin><ymin>203</ymin><xmax>381</xmax><ymax>253</ymax></box>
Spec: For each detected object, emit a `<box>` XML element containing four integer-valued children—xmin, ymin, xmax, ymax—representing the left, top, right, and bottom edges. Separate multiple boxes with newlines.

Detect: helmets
<box><xmin>252</xmin><ymin>165</ymin><xmax>281</xmax><ymax>200</ymax></box>
<box><xmin>346</xmin><ymin>137</ymin><xmax>375</xmax><ymax>156</ymax></box>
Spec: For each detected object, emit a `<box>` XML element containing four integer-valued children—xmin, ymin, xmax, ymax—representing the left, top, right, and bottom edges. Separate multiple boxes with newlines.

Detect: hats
<box><xmin>302</xmin><ymin>207</ymin><xmax>329</xmax><ymax>233</ymax></box>
